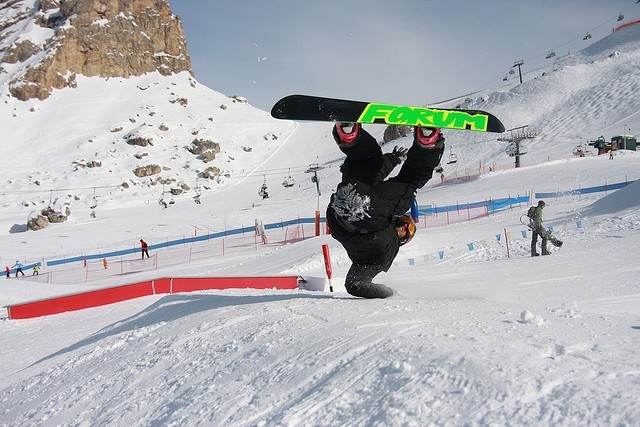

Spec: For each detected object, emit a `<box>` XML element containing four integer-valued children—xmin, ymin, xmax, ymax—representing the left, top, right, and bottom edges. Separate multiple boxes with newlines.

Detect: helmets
<box><xmin>395</xmin><ymin>213</ymin><xmax>417</xmax><ymax>247</ymax></box>
<box><xmin>538</xmin><ymin>201</ymin><xmax>545</xmax><ymax>206</ymax></box>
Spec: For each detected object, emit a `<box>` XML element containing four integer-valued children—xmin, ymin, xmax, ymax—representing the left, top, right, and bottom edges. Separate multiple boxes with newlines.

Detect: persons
<box><xmin>440</xmin><ymin>172</ymin><xmax>444</xmax><ymax>184</ymax></box>
<box><xmin>139</xmin><ymin>239</ymin><xmax>149</xmax><ymax>259</ymax></box>
<box><xmin>33</xmin><ymin>263</ymin><xmax>40</xmax><ymax>276</ymax></box>
<box><xmin>531</xmin><ymin>200</ymin><xmax>551</xmax><ymax>256</ymax></box>
<box><xmin>4</xmin><ymin>267</ymin><xmax>11</xmax><ymax>278</ymax></box>
<box><xmin>11</xmin><ymin>261</ymin><xmax>24</xmax><ymax>278</ymax></box>
<box><xmin>332</xmin><ymin>121</ymin><xmax>444</xmax><ymax>298</ymax></box>
<box><xmin>160</xmin><ymin>199</ymin><xmax>167</xmax><ymax>208</ymax></box>
<box><xmin>509</xmin><ymin>147</ymin><xmax>515</xmax><ymax>156</ymax></box>
<box><xmin>608</xmin><ymin>150</ymin><xmax>613</xmax><ymax>160</ymax></box>
<box><xmin>576</xmin><ymin>149</ymin><xmax>583</xmax><ymax>157</ymax></box>
<box><xmin>260</xmin><ymin>186</ymin><xmax>268</xmax><ymax>197</ymax></box>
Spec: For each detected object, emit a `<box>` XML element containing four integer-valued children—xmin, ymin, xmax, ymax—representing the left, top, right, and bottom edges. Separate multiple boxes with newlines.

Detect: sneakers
<box><xmin>542</xmin><ymin>250</ymin><xmax>552</xmax><ymax>256</ymax></box>
<box><xmin>531</xmin><ymin>252</ymin><xmax>540</xmax><ymax>257</ymax></box>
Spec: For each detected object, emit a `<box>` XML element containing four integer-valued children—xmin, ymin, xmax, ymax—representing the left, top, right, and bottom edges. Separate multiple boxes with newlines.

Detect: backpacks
<box><xmin>527</xmin><ymin>206</ymin><xmax>538</xmax><ymax>221</ymax></box>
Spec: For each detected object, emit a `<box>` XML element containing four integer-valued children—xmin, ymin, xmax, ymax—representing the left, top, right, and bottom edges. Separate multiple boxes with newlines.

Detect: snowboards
<box><xmin>520</xmin><ymin>214</ymin><xmax>563</xmax><ymax>247</ymax></box>
<box><xmin>271</xmin><ymin>95</ymin><xmax>505</xmax><ymax>133</ymax></box>
